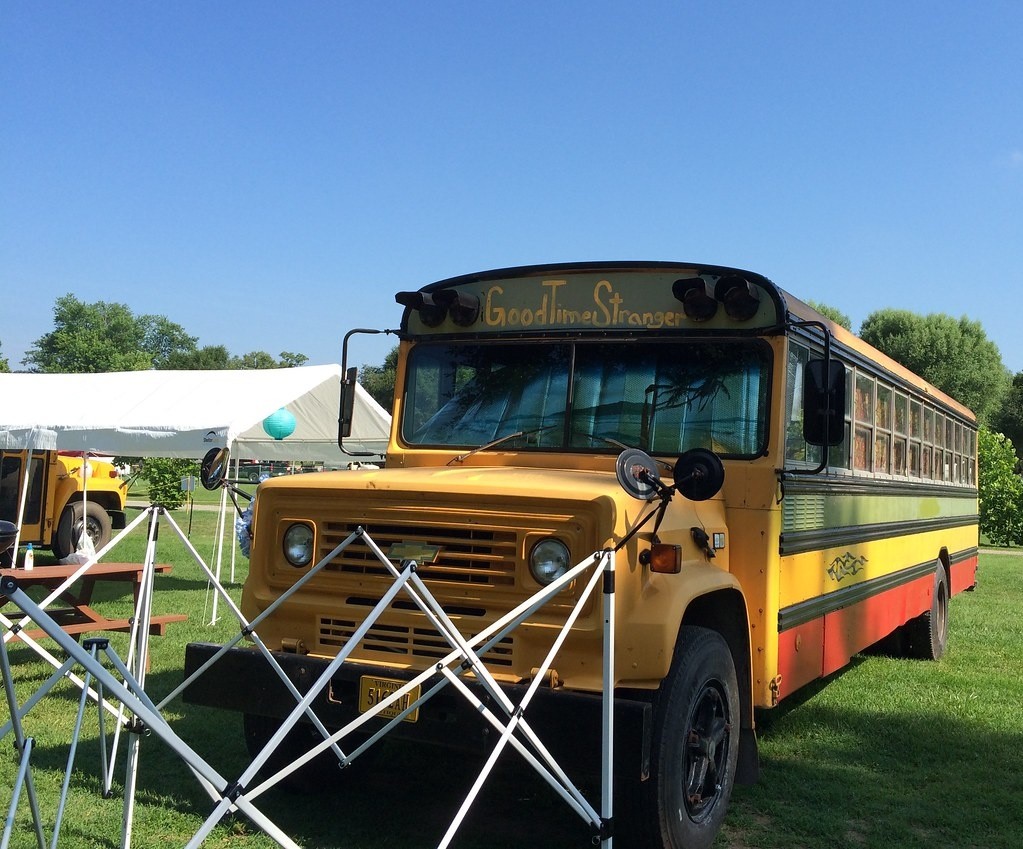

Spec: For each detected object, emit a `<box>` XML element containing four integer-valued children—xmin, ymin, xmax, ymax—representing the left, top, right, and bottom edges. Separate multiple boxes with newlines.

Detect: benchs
<box><xmin>0</xmin><ymin>615</ymin><xmax>188</xmax><ymax>674</ymax></box>
<box><xmin>1</xmin><ymin>609</ymin><xmax>75</xmax><ymax>625</ymax></box>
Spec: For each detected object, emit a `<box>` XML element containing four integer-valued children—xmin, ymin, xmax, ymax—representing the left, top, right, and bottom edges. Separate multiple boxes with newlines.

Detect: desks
<box><xmin>0</xmin><ymin>563</ymin><xmax>173</xmax><ymax>673</ymax></box>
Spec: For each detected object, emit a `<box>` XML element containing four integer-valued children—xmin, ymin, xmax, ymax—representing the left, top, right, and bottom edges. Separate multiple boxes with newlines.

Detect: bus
<box><xmin>180</xmin><ymin>259</ymin><xmax>984</xmax><ymax>849</ymax></box>
<box><xmin>229</xmin><ymin>458</ymin><xmax>290</xmax><ymax>481</ymax></box>
<box><xmin>0</xmin><ymin>450</ymin><xmax>130</xmax><ymax>566</ymax></box>
